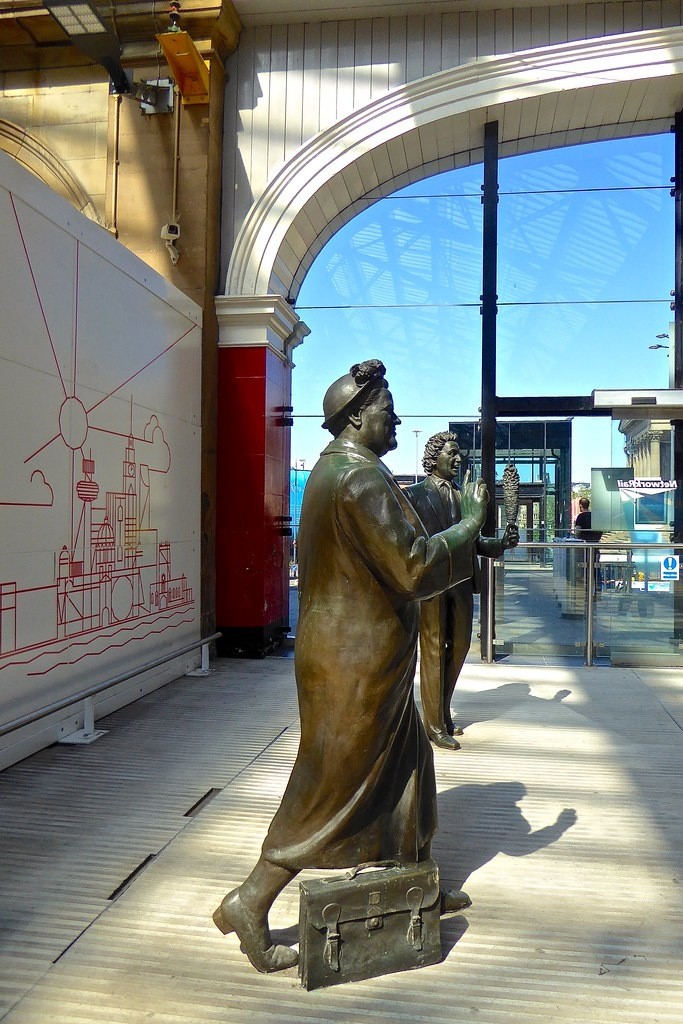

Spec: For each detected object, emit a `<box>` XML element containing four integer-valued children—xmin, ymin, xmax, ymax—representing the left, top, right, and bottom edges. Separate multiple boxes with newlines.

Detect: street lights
<box><xmin>300</xmin><ymin>458</ymin><xmax>306</xmax><ymax>470</ymax></box>
<box><xmin>411</xmin><ymin>430</ymin><xmax>423</xmax><ymax>484</ymax></box>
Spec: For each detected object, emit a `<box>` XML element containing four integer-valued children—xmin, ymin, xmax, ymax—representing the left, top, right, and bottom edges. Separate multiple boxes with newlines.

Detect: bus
<box><xmin>596</xmin><ymin>552</ymin><xmax>683</xmax><ymax>600</ymax></box>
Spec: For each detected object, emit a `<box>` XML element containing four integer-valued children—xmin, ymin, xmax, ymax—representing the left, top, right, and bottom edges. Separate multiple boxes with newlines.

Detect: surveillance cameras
<box><xmin>160</xmin><ymin>223</ymin><xmax>181</xmax><ymax>240</ymax></box>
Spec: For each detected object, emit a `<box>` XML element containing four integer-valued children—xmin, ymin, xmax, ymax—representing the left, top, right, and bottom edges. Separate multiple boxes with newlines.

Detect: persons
<box><xmin>575</xmin><ymin>498</ymin><xmax>603</xmax><ymax>603</ymax></box>
<box><xmin>210</xmin><ymin>359</ymin><xmax>489</xmax><ymax>975</ymax></box>
<box><xmin>399</xmin><ymin>431</ymin><xmax>520</xmax><ymax>750</ymax></box>
